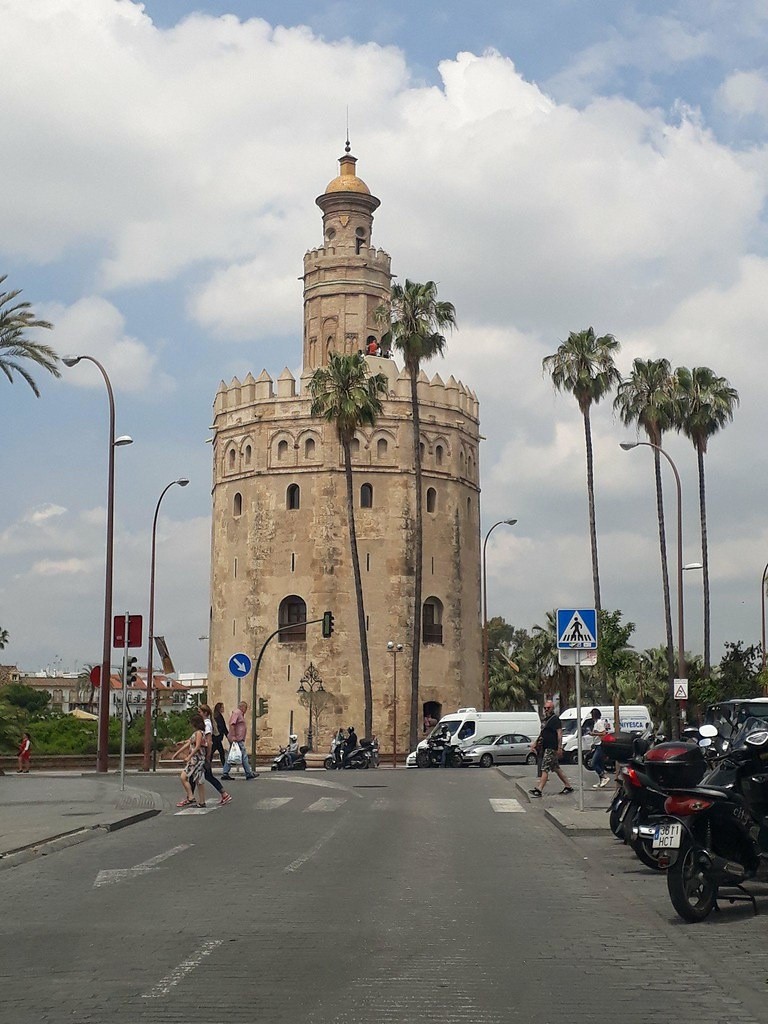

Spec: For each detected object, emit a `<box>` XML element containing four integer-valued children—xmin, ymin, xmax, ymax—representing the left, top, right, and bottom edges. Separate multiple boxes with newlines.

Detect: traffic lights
<box><xmin>321</xmin><ymin>611</ymin><xmax>335</xmax><ymax>639</ymax></box>
<box><xmin>122</xmin><ymin>656</ymin><xmax>138</xmax><ymax>686</ymax></box>
<box><xmin>257</xmin><ymin>696</ymin><xmax>269</xmax><ymax>717</ymax></box>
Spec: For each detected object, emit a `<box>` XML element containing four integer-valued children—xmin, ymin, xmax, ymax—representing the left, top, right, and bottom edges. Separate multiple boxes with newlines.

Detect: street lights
<box><xmin>295</xmin><ymin>661</ymin><xmax>327</xmax><ymax>754</ymax></box>
<box><xmin>617</xmin><ymin>437</ymin><xmax>703</xmax><ymax>736</ymax></box>
<box><xmin>142</xmin><ymin>476</ymin><xmax>190</xmax><ymax>771</ymax></box>
<box><xmin>482</xmin><ymin>518</ymin><xmax>518</xmax><ymax>712</ymax></box>
<box><xmin>60</xmin><ymin>354</ymin><xmax>135</xmax><ymax>773</ymax></box>
<box><xmin>383</xmin><ymin>639</ymin><xmax>405</xmax><ymax>769</ymax></box>
<box><xmin>638</xmin><ymin>656</ymin><xmax>649</xmax><ymax>706</ymax></box>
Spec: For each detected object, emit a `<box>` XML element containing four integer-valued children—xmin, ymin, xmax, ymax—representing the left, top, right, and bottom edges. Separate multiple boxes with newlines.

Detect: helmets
<box><xmin>347</xmin><ymin>727</ymin><xmax>354</xmax><ymax>732</ymax></box>
<box><xmin>441</xmin><ymin>724</ymin><xmax>448</xmax><ymax>728</ymax></box>
<box><xmin>289</xmin><ymin>735</ymin><xmax>297</xmax><ymax>740</ymax></box>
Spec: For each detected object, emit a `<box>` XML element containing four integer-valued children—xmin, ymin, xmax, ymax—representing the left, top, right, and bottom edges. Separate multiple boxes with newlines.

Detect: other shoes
<box><xmin>221</xmin><ymin>776</ymin><xmax>235</xmax><ymax>780</ymax></box>
<box><xmin>246</xmin><ymin>773</ymin><xmax>260</xmax><ymax>780</ymax></box>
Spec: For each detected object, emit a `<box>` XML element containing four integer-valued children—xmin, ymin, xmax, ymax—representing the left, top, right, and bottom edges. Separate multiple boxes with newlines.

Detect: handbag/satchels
<box><xmin>226</xmin><ymin>741</ymin><xmax>242</xmax><ymax>765</ymax></box>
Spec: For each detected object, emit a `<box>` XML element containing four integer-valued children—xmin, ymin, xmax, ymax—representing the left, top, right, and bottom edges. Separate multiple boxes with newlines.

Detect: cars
<box><xmin>461</xmin><ymin>733</ymin><xmax>538</xmax><ymax>769</ymax></box>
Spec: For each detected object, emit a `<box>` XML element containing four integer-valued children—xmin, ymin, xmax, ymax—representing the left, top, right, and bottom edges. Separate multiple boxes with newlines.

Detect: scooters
<box><xmin>270</xmin><ymin>745</ymin><xmax>309</xmax><ymax>771</ymax></box>
<box><xmin>324</xmin><ymin>736</ymin><xmax>381</xmax><ymax>770</ymax></box>
<box><xmin>415</xmin><ymin>733</ymin><xmax>465</xmax><ymax>767</ymax></box>
<box><xmin>584</xmin><ymin>707</ymin><xmax>768</xmax><ymax>923</ymax></box>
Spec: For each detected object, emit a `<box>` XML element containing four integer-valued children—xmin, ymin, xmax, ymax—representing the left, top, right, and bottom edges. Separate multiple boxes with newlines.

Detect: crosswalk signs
<box><xmin>556</xmin><ymin>609</ymin><xmax>598</xmax><ymax>650</ymax></box>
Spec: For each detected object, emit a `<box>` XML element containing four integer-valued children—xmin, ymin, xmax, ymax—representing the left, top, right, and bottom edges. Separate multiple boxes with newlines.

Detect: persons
<box><xmin>220</xmin><ymin>701</ymin><xmax>260</xmax><ymax>780</ymax></box>
<box><xmin>588</xmin><ymin>709</ymin><xmax>610</xmax><ymax>788</ymax></box>
<box><xmin>434</xmin><ymin>724</ymin><xmax>452</xmax><ymax>768</ymax></box>
<box><xmin>285</xmin><ymin>735</ymin><xmax>299</xmax><ymax>766</ymax></box>
<box><xmin>357</xmin><ymin>340</ymin><xmax>393</xmax><ymax>361</ymax></box>
<box><xmin>187</xmin><ymin>704</ymin><xmax>232</xmax><ymax>805</ymax></box>
<box><xmin>528</xmin><ymin>700</ymin><xmax>574</xmax><ymax>797</ymax></box>
<box><xmin>208</xmin><ymin>703</ymin><xmax>229</xmax><ymax>766</ymax></box>
<box><xmin>172</xmin><ymin>714</ymin><xmax>207</xmax><ymax>808</ymax></box>
<box><xmin>341</xmin><ymin>726</ymin><xmax>357</xmax><ymax>770</ymax></box>
<box><xmin>17</xmin><ymin>733</ymin><xmax>31</xmax><ymax>773</ymax></box>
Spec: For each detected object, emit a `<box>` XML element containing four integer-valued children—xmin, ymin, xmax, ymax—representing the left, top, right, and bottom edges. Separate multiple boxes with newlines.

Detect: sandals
<box><xmin>192</xmin><ymin>803</ymin><xmax>206</xmax><ymax>808</ymax></box>
<box><xmin>182</xmin><ymin>796</ymin><xmax>196</xmax><ymax>805</ymax></box>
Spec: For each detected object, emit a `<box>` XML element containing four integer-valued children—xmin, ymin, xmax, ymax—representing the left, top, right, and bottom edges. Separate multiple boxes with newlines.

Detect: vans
<box><xmin>415</xmin><ymin>708</ymin><xmax>543</xmax><ymax>768</ymax></box>
<box><xmin>558</xmin><ymin>704</ymin><xmax>654</xmax><ymax>765</ymax></box>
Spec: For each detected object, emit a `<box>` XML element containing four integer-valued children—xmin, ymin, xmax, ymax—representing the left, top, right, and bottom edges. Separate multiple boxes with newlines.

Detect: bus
<box><xmin>704</xmin><ymin>696</ymin><xmax>768</xmax><ymax>737</ymax></box>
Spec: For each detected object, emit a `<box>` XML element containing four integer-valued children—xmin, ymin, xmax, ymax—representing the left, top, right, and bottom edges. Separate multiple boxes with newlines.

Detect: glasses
<box><xmin>544</xmin><ymin>707</ymin><xmax>551</xmax><ymax>710</ymax></box>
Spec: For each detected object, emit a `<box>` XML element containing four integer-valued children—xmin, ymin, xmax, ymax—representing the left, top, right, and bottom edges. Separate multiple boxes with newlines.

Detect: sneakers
<box><xmin>592</xmin><ymin>782</ymin><xmax>600</xmax><ymax>788</ymax></box>
<box><xmin>218</xmin><ymin>794</ymin><xmax>232</xmax><ymax>804</ymax></box>
<box><xmin>529</xmin><ymin>787</ymin><xmax>543</xmax><ymax>797</ymax></box>
<box><xmin>600</xmin><ymin>777</ymin><xmax>610</xmax><ymax>787</ymax></box>
<box><xmin>559</xmin><ymin>787</ymin><xmax>574</xmax><ymax>794</ymax></box>
<box><xmin>176</xmin><ymin>800</ymin><xmax>184</xmax><ymax>806</ymax></box>
